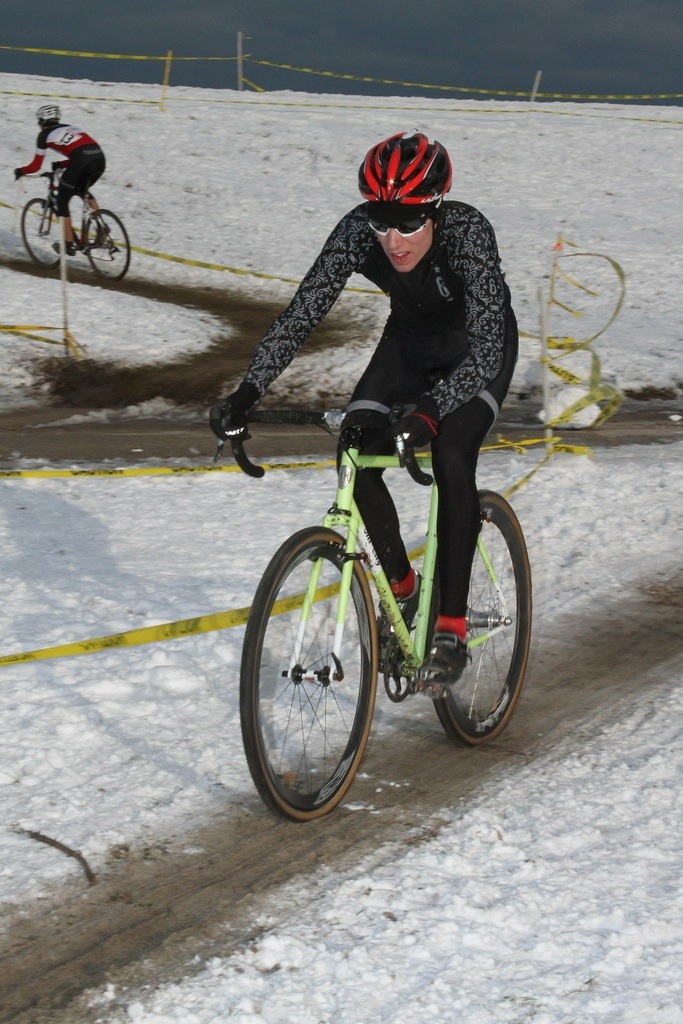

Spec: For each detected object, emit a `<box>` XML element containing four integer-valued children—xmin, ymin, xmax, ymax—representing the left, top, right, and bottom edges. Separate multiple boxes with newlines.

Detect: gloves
<box><xmin>210</xmin><ymin>385</ymin><xmax>258</xmax><ymax>440</ymax></box>
<box><xmin>14</xmin><ymin>169</ymin><xmax>25</xmax><ymax>179</ymax></box>
<box><xmin>52</xmin><ymin>162</ymin><xmax>61</xmax><ymax>170</ymax></box>
<box><xmin>390</xmin><ymin>396</ymin><xmax>440</xmax><ymax>449</ymax></box>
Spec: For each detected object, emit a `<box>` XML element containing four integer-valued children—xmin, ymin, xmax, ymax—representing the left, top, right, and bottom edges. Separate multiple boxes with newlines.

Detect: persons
<box><xmin>14</xmin><ymin>104</ymin><xmax>109</xmax><ymax>255</ymax></box>
<box><xmin>209</xmin><ymin>127</ymin><xmax>519</xmax><ymax>683</ymax></box>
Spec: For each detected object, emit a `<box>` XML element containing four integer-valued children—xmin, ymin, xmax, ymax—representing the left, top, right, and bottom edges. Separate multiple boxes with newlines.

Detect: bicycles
<box><xmin>14</xmin><ymin>161</ymin><xmax>132</xmax><ymax>282</ymax></box>
<box><xmin>213</xmin><ymin>403</ymin><xmax>534</xmax><ymax>823</ymax></box>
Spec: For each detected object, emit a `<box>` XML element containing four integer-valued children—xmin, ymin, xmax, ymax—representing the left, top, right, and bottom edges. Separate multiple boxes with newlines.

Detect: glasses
<box><xmin>367</xmin><ymin>215</ymin><xmax>429</xmax><ymax>237</ymax></box>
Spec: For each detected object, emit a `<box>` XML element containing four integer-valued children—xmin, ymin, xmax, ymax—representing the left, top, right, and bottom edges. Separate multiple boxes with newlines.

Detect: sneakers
<box><xmin>52</xmin><ymin>241</ymin><xmax>76</xmax><ymax>256</ymax></box>
<box><xmin>420</xmin><ymin>626</ymin><xmax>473</xmax><ymax>683</ymax></box>
<box><xmin>94</xmin><ymin>224</ymin><xmax>110</xmax><ymax>248</ymax></box>
<box><xmin>381</xmin><ymin>571</ymin><xmax>421</xmax><ymax>651</ymax></box>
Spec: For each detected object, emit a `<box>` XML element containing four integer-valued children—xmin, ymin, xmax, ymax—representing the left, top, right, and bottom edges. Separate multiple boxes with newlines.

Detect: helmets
<box><xmin>36</xmin><ymin>106</ymin><xmax>60</xmax><ymax>122</ymax></box>
<box><xmin>358</xmin><ymin>130</ymin><xmax>452</xmax><ymax>222</ymax></box>
